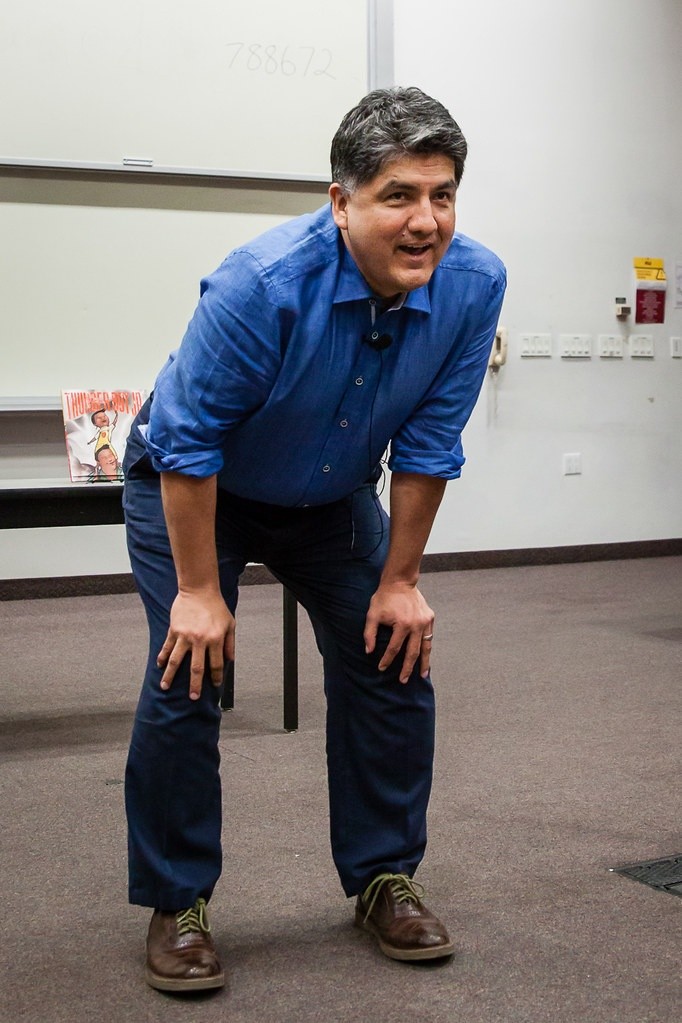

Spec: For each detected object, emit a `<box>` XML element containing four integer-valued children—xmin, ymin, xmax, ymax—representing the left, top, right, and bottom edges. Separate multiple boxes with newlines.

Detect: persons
<box><xmin>121</xmin><ymin>86</ymin><xmax>508</xmax><ymax>993</ymax></box>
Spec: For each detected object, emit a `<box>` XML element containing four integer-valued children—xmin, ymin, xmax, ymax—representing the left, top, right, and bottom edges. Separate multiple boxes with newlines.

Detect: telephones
<box><xmin>489</xmin><ymin>328</ymin><xmax>509</xmax><ymax>369</ymax></box>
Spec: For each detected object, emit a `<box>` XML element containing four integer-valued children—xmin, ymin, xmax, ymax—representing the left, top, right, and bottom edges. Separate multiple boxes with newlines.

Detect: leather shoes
<box><xmin>146</xmin><ymin>896</ymin><xmax>226</xmax><ymax>994</ymax></box>
<box><xmin>353</xmin><ymin>872</ymin><xmax>455</xmax><ymax>962</ymax></box>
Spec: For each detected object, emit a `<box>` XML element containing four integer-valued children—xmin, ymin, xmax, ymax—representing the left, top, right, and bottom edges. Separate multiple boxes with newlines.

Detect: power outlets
<box><xmin>559</xmin><ymin>333</ymin><xmax>592</xmax><ymax>359</ymax></box>
<box><xmin>670</xmin><ymin>336</ymin><xmax>682</xmax><ymax>359</ymax></box>
<box><xmin>519</xmin><ymin>332</ymin><xmax>552</xmax><ymax>358</ymax></box>
<box><xmin>629</xmin><ymin>334</ymin><xmax>654</xmax><ymax>357</ymax></box>
<box><xmin>597</xmin><ymin>334</ymin><xmax>624</xmax><ymax>358</ymax></box>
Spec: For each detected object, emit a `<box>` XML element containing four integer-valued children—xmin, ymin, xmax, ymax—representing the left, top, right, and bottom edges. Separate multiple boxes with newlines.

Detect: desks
<box><xmin>0</xmin><ymin>475</ymin><xmax>298</xmax><ymax>733</ymax></box>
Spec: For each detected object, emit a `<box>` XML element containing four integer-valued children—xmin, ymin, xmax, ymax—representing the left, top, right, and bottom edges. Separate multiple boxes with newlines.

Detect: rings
<box><xmin>421</xmin><ymin>634</ymin><xmax>433</xmax><ymax>641</ymax></box>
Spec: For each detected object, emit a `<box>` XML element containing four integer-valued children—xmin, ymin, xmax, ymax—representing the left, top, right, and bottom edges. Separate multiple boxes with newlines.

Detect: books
<box><xmin>62</xmin><ymin>389</ymin><xmax>144</xmax><ymax>483</ymax></box>
